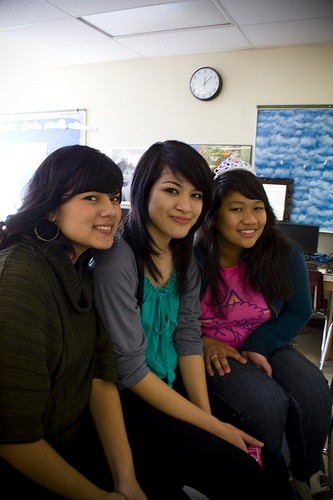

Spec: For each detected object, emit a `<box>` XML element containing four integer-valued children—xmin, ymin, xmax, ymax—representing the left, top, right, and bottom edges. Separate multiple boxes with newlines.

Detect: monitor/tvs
<box><xmin>277</xmin><ymin>222</ymin><xmax>319</xmax><ymax>256</ymax></box>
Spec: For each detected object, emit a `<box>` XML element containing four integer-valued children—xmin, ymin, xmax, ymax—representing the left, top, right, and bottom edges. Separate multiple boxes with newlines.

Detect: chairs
<box><xmin>308</xmin><ymin>270</ymin><xmax>327</xmax><ymax>364</ymax></box>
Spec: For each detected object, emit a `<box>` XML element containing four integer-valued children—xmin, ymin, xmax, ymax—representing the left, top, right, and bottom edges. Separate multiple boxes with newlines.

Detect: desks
<box><xmin>306</xmin><ymin>261</ymin><xmax>333</xmax><ymax>361</ymax></box>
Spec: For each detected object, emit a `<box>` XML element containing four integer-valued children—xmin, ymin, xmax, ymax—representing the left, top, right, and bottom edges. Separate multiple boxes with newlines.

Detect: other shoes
<box><xmin>288</xmin><ymin>470</ymin><xmax>333</xmax><ymax>500</ymax></box>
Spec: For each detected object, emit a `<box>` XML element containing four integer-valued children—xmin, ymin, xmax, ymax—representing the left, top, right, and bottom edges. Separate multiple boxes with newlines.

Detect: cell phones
<box><xmin>247</xmin><ymin>446</ymin><xmax>263</xmax><ymax>469</ymax></box>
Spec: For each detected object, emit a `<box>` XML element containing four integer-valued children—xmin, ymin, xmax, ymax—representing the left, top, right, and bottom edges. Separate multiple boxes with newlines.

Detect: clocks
<box><xmin>189</xmin><ymin>67</ymin><xmax>223</xmax><ymax>101</ymax></box>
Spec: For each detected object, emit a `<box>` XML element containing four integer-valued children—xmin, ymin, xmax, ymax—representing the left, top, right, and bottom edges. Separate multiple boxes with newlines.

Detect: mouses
<box><xmin>326</xmin><ymin>268</ymin><xmax>333</xmax><ymax>273</ymax></box>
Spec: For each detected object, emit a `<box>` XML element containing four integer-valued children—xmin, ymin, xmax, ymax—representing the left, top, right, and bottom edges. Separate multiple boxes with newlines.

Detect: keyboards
<box><xmin>306</xmin><ymin>261</ymin><xmax>321</xmax><ymax>270</ymax></box>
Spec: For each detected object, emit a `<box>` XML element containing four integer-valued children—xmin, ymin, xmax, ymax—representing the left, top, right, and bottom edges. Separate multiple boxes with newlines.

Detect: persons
<box><xmin>90</xmin><ymin>139</ymin><xmax>297</xmax><ymax>500</ymax></box>
<box><xmin>0</xmin><ymin>146</ymin><xmax>147</xmax><ymax>500</ymax></box>
<box><xmin>194</xmin><ymin>163</ymin><xmax>333</xmax><ymax>500</ymax></box>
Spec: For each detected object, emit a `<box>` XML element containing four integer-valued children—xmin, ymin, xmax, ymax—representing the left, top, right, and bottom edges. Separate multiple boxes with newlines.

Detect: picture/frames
<box><xmin>256</xmin><ymin>176</ymin><xmax>294</xmax><ymax>223</ymax></box>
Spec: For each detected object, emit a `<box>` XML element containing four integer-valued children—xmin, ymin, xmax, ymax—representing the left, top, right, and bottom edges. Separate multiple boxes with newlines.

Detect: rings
<box><xmin>210</xmin><ymin>355</ymin><xmax>218</xmax><ymax>360</ymax></box>
